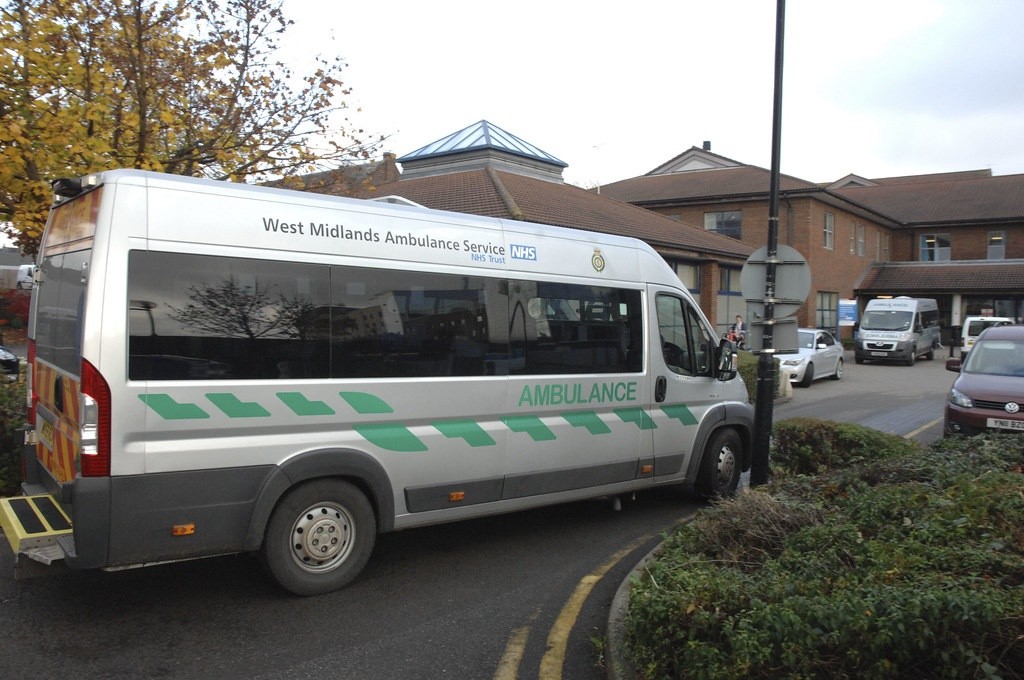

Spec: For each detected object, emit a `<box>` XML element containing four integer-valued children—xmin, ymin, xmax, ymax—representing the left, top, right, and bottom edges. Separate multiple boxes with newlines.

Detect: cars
<box><xmin>773</xmin><ymin>327</ymin><xmax>844</xmax><ymax>387</ymax></box>
<box><xmin>944</xmin><ymin>321</ymin><xmax>1024</xmax><ymax>438</ymax></box>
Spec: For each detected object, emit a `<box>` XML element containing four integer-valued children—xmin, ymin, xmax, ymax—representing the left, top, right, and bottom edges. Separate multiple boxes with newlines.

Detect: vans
<box><xmin>853</xmin><ymin>296</ymin><xmax>940</xmax><ymax>366</ymax></box>
<box><xmin>2</xmin><ymin>168</ymin><xmax>755</xmax><ymax>597</ymax></box>
<box><xmin>16</xmin><ymin>264</ymin><xmax>36</xmax><ymax>289</ymax></box>
<box><xmin>960</xmin><ymin>317</ymin><xmax>1016</xmax><ymax>365</ymax></box>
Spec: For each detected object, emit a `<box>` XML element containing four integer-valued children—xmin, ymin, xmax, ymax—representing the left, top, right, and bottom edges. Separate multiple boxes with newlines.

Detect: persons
<box><xmin>732</xmin><ymin>315</ymin><xmax>747</xmax><ymax>348</ymax></box>
